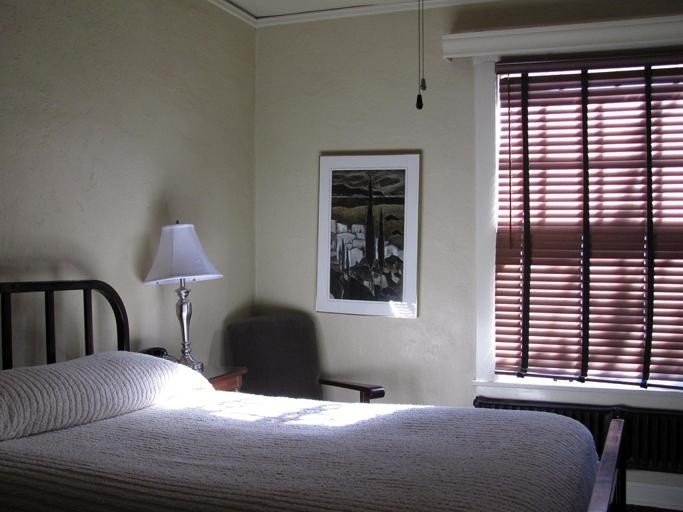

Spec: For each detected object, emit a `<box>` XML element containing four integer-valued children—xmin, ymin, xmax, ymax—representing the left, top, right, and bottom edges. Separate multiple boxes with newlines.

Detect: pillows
<box><xmin>0</xmin><ymin>347</ymin><xmax>215</xmax><ymax>442</ymax></box>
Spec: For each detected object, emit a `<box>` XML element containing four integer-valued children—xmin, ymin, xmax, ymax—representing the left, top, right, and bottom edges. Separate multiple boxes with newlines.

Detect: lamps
<box><xmin>143</xmin><ymin>218</ymin><xmax>225</xmax><ymax>371</ymax></box>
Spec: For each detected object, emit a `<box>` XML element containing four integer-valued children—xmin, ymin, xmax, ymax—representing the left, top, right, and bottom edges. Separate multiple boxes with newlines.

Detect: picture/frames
<box><xmin>312</xmin><ymin>151</ymin><xmax>423</xmax><ymax>320</ymax></box>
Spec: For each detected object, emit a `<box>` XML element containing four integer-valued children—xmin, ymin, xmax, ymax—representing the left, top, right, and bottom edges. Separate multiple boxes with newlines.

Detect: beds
<box><xmin>0</xmin><ymin>279</ymin><xmax>628</xmax><ymax>512</ymax></box>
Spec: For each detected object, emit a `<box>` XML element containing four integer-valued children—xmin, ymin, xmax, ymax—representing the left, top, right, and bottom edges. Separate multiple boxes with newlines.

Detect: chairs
<box><xmin>227</xmin><ymin>313</ymin><xmax>386</xmax><ymax>402</ymax></box>
